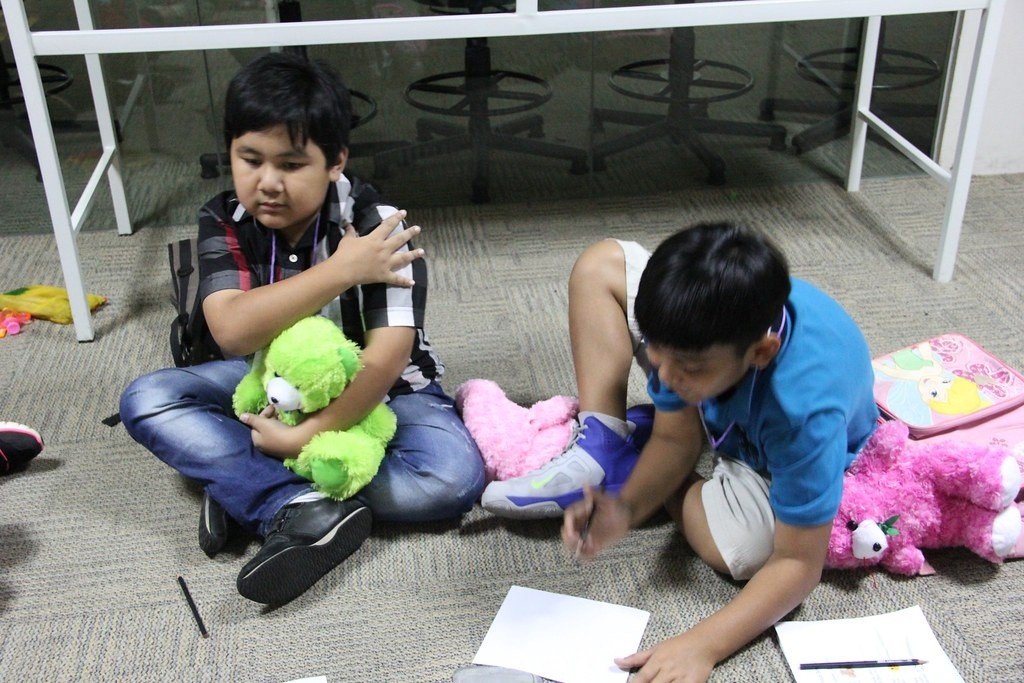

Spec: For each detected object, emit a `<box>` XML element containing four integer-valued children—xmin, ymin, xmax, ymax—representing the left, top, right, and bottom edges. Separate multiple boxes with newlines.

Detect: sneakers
<box><xmin>236</xmin><ymin>495</ymin><xmax>375</xmax><ymax>606</ymax></box>
<box><xmin>479</xmin><ymin>416</ymin><xmax>643</xmax><ymax>521</ymax></box>
<box><xmin>0</xmin><ymin>421</ymin><xmax>44</xmax><ymax>478</ymax></box>
<box><xmin>197</xmin><ymin>488</ymin><xmax>238</xmax><ymax>559</ymax></box>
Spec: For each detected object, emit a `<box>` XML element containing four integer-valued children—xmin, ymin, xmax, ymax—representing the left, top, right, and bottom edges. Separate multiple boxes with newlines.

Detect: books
<box><xmin>773</xmin><ymin>603</ymin><xmax>967</xmax><ymax>683</ymax></box>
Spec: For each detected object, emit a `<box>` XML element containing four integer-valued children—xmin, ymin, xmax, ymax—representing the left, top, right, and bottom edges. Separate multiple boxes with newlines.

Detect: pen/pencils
<box><xmin>576</xmin><ymin>478</ymin><xmax>607</xmax><ymax>561</ymax></box>
<box><xmin>801</xmin><ymin>657</ymin><xmax>929</xmax><ymax>669</ymax></box>
<box><xmin>177</xmin><ymin>575</ymin><xmax>210</xmax><ymax>638</ymax></box>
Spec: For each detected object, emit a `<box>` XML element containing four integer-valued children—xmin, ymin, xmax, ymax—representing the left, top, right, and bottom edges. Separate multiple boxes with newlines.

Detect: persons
<box><xmin>557</xmin><ymin>219</ymin><xmax>882</xmax><ymax>683</ymax></box>
<box><xmin>117</xmin><ymin>51</ymin><xmax>487</xmax><ymax>608</ymax></box>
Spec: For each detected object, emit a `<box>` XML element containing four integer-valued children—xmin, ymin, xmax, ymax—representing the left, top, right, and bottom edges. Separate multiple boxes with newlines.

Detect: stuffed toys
<box><xmin>820</xmin><ymin>418</ymin><xmax>1024</xmax><ymax>577</ymax></box>
<box><xmin>231</xmin><ymin>311</ymin><xmax>397</xmax><ymax>502</ymax></box>
<box><xmin>455</xmin><ymin>378</ymin><xmax>579</xmax><ymax>482</ymax></box>
